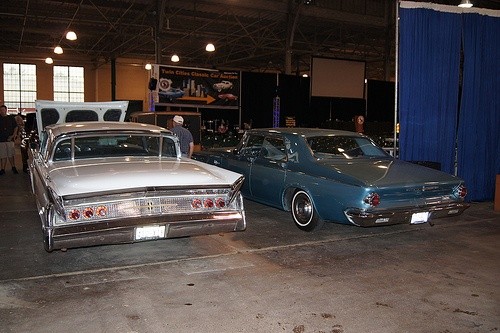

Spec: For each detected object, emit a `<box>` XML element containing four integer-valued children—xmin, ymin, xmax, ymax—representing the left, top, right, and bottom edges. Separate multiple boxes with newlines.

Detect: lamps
<box><xmin>43</xmin><ymin>49</ymin><xmax>54</xmax><ymax>64</ymax></box>
<box><xmin>205</xmin><ymin>37</ymin><xmax>215</xmax><ymax>52</ymax></box>
<box><xmin>53</xmin><ymin>42</ymin><xmax>65</xmax><ymax>54</ymax></box>
<box><xmin>144</xmin><ymin>61</ymin><xmax>153</xmax><ymax>70</ymax></box>
<box><xmin>171</xmin><ymin>50</ymin><xmax>180</xmax><ymax>62</ymax></box>
<box><xmin>66</xmin><ymin>25</ymin><xmax>78</xmax><ymax>41</ymax></box>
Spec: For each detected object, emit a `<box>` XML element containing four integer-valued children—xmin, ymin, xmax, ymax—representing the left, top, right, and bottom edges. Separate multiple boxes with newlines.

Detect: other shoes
<box><xmin>0</xmin><ymin>169</ymin><xmax>5</xmax><ymax>176</ymax></box>
<box><xmin>12</xmin><ymin>167</ymin><xmax>18</xmax><ymax>175</ymax></box>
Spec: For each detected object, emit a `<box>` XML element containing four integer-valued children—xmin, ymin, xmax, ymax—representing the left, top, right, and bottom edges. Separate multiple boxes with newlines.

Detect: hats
<box><xmin>173</xmin><ymin>115</ymin><xmax>184</xmax><ymax>124</ymax></box>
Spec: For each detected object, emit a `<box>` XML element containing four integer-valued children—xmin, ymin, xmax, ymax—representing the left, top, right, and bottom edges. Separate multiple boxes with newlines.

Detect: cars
<box><xmin>219</xmin><ymin>93</ymin><xmax>237</xmax><ymax>101</ymax></box>
<box><xmin>214</xmin><ymin>81</ymin><xmax>232</xmax><ymax>90</ymax></box>
<box><xmin>191</xmin><ymin>126</ymin><xmax>471</xmax><ymax>232</ymax></box>
<box><xmin>27</xmin><ymin>120</ymin><xmax>248</xmax><ymax>254</ymax></box>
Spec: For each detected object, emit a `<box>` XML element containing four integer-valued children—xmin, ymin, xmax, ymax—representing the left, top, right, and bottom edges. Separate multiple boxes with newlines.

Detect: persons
<box><xmin>170</xmin><ymin>114</ymin><xmax>194</xmax><ymax>159</ymax></box>
<box><xmin>20</xmin><ymin>128</ymin><xmax>39</xmax><ymax>172</ymax></box>
<box><xmin>0</xmin><ymin>105</ymin><xmax>19</xmax><ymax>175</ymax></box>
